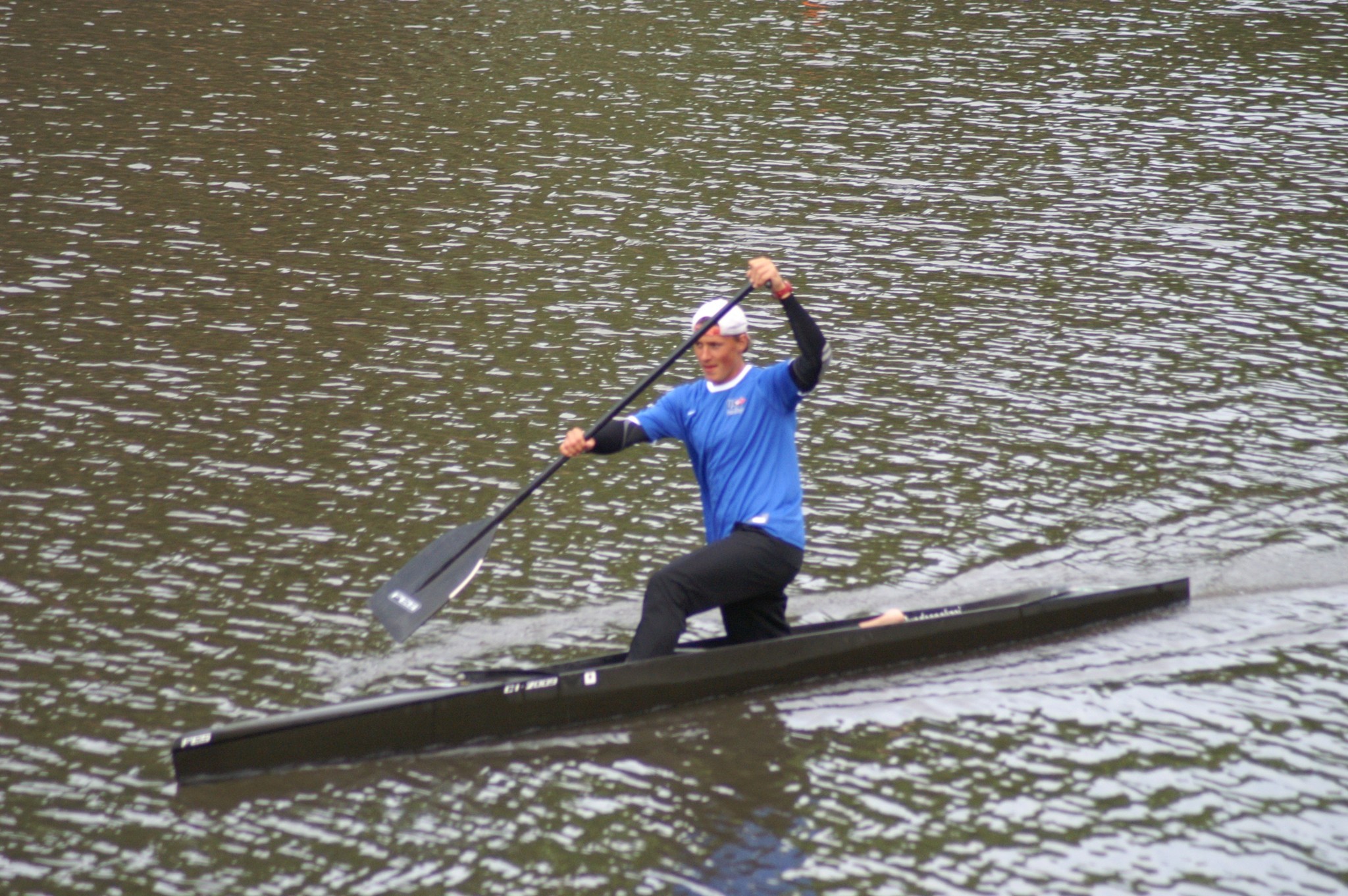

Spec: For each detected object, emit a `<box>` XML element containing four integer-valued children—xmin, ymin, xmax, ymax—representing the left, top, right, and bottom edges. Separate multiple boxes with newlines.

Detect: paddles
<box><xmin>367</xmin><ymin>274</ymin><xmax>775</xmax><ymax>644</ymax></box>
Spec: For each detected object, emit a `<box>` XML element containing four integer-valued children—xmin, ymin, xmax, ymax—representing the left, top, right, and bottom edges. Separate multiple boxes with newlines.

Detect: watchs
<box><xmin>773</xmin><ymin>279</ymin><xmax>792</xmax><ymax>298</ymax></box>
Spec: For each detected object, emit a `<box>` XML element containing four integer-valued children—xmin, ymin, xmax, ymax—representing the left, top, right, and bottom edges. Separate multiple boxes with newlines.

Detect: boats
<box><xmin>172</xmin><ymin>578</ymin><xmax>1190</xmax><ymax>776</ymax></box>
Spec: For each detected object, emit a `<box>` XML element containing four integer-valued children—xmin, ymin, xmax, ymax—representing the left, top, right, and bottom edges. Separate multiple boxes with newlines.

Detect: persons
<box><xmin>559</xmin><ymin>256</ymin><xmax>832</xmax><ymax>661</ymax></box>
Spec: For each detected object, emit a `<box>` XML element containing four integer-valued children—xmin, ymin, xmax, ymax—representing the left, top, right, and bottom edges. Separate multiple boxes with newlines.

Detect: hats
<box><xmin>692</xmin><ymin>298</ymin><xmax>748</xmax><ymax>336</ymax></box>
